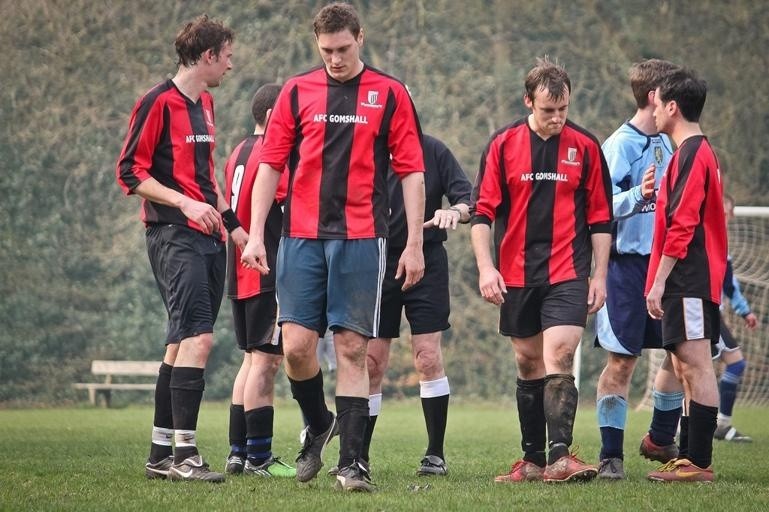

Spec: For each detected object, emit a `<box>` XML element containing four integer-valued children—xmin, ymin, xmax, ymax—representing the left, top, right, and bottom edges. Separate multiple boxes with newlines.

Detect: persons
<box><xmin>327</xmin><ymin>82</ymin><xmax>473</xmax><ymax>478</ymax></box>
<box><xmin>468</xmin><ymin>52</ymin><xmax>615</xmax><ymax>481</ymax></box>
<box><xmin>226</xmin><ymin>82</ymin><xmax>300</xmax><ymax>480</ymax></box>
<box><xmin>238</xmin><ymin>2</ymin><xmax>427</xmax><ymax>490</ymax></box>
<box><xmin>114</xmin><ymin>13</ymin><xmax>251</xmax><ymax>487</ymax></box>
<box><xmin>710</xmin><ymin>193</ymin><xmax>761</xmax><ymax>443</ymax></box>
<box><xmin>645</xmin><ymin>67</ymin><xmax>728</xmax><ymax>485</ymax></box>
<box><xmin>594</xmin><ymin>57</ymin><xmax>682</xmax><ymax>482</ymax></box>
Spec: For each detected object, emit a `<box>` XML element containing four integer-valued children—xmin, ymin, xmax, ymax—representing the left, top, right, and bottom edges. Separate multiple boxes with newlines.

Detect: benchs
<box><xmin>70</xmin><ymin>359</ymin><xmax>162</xmax><ymax>407</ymax></box>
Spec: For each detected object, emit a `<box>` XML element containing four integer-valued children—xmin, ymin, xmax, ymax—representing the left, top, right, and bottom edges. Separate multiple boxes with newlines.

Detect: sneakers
<box><xmin>297</xmin><ymin>410</ymin><xmax>339</xmax><ymax>482</ymax></box>
<box><xmin>146</xmin><ymin>457</ymin><xmax>174</xmax><ymax>479</ymax></box>
<box><xmin>167</xmin><ymin>455</ymin><xmax>225</xmax><ymax>482</ymax></box>
<box><xmin>598</xmin><ymin>458</ymin><xmax>623</xmax><ymax>480</ymax></box>
<box><xmin>416</xmin><ymin>455</ymin><xmax>447</xmax><ymax>476</ymax></box>
<box><xmin>495</xmin><ymin>459</ymin><xmax>543</xmax><ymax>482</ymax></box>
<box><xmin>646</xmin><ymin>457</ymin><xmax>713</xmax><ymax>483</ymax></box>
<box><xmin>333</xmin><ymin>462</ymin><xmax>373</xmax><ymax>492</ymax></box>
<box><xmin>243</xmin><ymin>452</ymin><xmax>296</xmax><ymax>478</ymax></box>
<box><xmin>713</xmin><ymin>424</ymin><xmax>752</xmax><ymax>442</ymax></box>
<box><xmin>225</xmin><ymin>451</ymin><xmax>244</xmax><ymax>475</ymax></box>
<box><xmin>327</xmin><ymin>457</ymin><xmax>371</xmax><ymax>476</ymax></box>
<box><xmin>639</xmin><ymin>433</ymin><xmax>679</xmax><ymax>464</ymax></box>
<box><xmin>543</xmin><ymin>454</ymin><xmax>598</xmax><ymax>483</ymax></box>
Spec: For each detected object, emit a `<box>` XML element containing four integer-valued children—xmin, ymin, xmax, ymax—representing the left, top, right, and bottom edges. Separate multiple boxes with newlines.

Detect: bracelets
<box><xmin>446</xmin><ymin>206</ymin><xmax>463</xmax><ymax>219</ymax></box>
<box><xmin>220</xmin><ymin>207</ymin><xmax>242</xmax><ymax>233</ymax></box>
